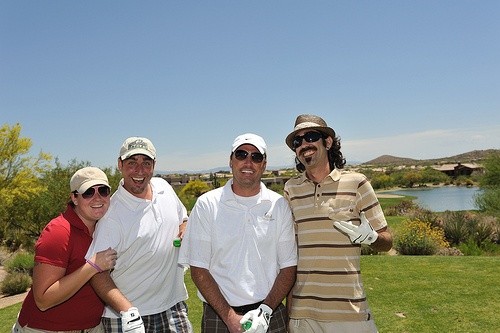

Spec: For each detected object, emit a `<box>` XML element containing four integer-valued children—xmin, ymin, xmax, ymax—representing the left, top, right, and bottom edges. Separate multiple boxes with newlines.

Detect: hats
<box><xmin>120</xmin><ymin>137</ymin><xmax>156</xmax><ymax>160</ymax></box>
<box><xmin>285</xmin><ymin>115</ymin><xmax>336</xmax><ymax>151</ymax></box>
<box><xmin>232</xmin><ymin>133</ymin><xmax>268</xmax><ymax>163</ymax></box>
<box><xmin>70</xmin><ymin>167</ymin><xmax>111</xmax><ymax>194</ymax></box>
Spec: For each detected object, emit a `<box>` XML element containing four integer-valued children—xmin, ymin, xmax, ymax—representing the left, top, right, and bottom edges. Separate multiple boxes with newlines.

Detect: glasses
<box><xmin>74</xmin><ymin>186</ymin><xmax>110</xmax><ymax>199</ymax></box>
<box><xmin>292</xmin><ymin>131</ymin><xmax>324</xmax><ymax>148</ymax></box>
<box><xmin>234</xmin><ymin>150</ymin><xmax>266</xmax><ymax>163</ymax></box>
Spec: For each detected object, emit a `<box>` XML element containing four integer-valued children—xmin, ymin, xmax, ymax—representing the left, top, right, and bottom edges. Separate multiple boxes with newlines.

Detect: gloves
<box><xmin>240</xmin><ymin>304</ymin><xmax>273</xmax><ymax>333</ymax></box>
<box><xmin>333</xmin><ymin>212</ymin><xmax>378</xmax><ymax>245</ymax></box>
<box><xmin>120</xmin><ymin>307</ymin><xmax>145</xmax><ymax>333</ymax></box>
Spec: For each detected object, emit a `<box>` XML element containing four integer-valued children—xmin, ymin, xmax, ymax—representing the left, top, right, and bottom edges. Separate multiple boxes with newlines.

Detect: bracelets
<box><xmin>86</xmin><ymin>260</ymin><xmax>102</xmax><ymax>273</ymax></box>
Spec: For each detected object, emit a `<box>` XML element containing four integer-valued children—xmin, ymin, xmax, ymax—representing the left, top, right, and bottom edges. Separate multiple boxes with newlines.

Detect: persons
<box><xmin>283</xmin><ymin>114</ymin><xmax>393</xmax><ymax>333</ymax></box>
<box><xmin>178</xmin><ymin>133</ymin><xmax>298</xmax><ymax>333</ymax></box>
<box><xmin>12</xmin><ymin>167</ymin><xmax>117</xmax><ymax>333</ymax></box>
<box><xmin>84</xmin><ymin>137</ymin><xmax>189</xmax><ymax>333</ymax></box>
